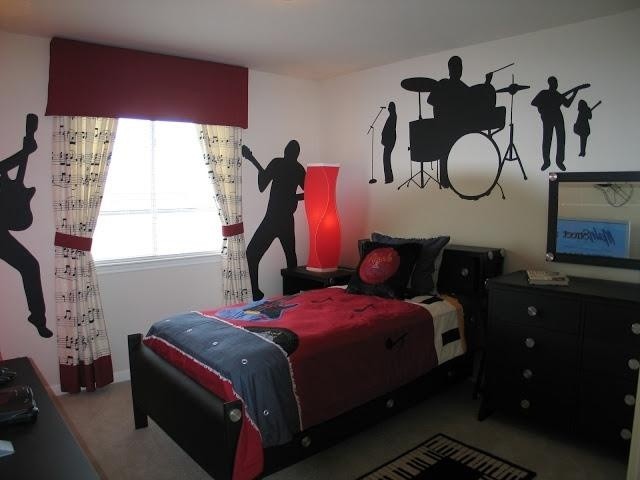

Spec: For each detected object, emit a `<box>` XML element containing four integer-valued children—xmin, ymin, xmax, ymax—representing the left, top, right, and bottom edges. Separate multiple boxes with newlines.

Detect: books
<box><xmin>527</xmin><ymin>269</ymin><xmax>569</xmax><ymax>287</ymax></box>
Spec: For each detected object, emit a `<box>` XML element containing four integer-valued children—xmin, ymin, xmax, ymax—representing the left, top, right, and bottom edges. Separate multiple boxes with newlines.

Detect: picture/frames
<box><xmin>546</xmin><ymin>170</ymin><xmax>640</xmax><ymax>269</ymax></box>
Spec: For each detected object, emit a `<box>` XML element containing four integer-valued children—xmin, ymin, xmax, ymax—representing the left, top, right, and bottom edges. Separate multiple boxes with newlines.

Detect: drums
<box><xmin>446</xmin><ymin>131</ymin><xmax>501</xmax><ymax>200</ymax></box>
<box><xmin>409</xmin><ymin>118</ymin><xmax>440</xmax><ymax>162</ymax></box>
<box><xmin>482</xmin><ymin>106</ymin><xmax>505</xmax><ymax>130</ymax></box>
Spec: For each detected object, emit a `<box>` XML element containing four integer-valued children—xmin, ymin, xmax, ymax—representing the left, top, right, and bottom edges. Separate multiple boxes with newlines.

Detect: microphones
<box><xmin>380</xmin><ymin>107</ymin><xmax>386</xmax><ymax>108</ymax></box>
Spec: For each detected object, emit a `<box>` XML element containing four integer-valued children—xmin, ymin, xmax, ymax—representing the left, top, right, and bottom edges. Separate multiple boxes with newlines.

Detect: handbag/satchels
<box><xmin>0</xmin><ymin>385</ymin><xmax>39</xmax><ymax>427</ymax></box>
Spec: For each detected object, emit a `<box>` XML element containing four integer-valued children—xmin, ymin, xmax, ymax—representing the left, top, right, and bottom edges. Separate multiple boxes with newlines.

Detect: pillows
<box><xmin>346</xmin><ymin>232</ymin><xmax>451</xmax><ymax>300</ymax></box>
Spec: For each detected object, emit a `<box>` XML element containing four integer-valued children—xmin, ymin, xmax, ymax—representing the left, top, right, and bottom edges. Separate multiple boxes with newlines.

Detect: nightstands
<box><xmin>281</xmin><ymin>265</ymin><xmax>358</xmax><ymax>296</ymax></box>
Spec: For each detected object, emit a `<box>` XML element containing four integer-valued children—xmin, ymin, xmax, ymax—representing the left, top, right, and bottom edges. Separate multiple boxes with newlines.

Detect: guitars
<box><xmin>0</xmin><ymin>113</ymin><xmax>38</xmax><ymax>231</ymax></box>
<box><xmin>242</xmin><ymin>145</ymin><xmax>297</xmax><ymax>213</ymax></box>
<box><xmin>213</xmin><ymin>292</ymin><xmax>303</xmax><ymax>321</ymax></box>
<box><xmin>593</xmin><ymin>184</ymin><xmax>632</xmax><ymax>207</ymax></box>
<box><xmin>574</xmin><ymin>101</ymin><xmax>601</xmax><ymax>136</ymax></box>
<box><xmin>538</xmin><ymin>83</ymin><xmax>590</xmax><ymax>113</ymax></box>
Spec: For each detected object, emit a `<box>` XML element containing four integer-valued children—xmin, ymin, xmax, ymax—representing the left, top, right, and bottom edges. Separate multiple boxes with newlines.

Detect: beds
<box><xmin>127</xmin><ymin>245</ymin><xmax>506</xmax><ymax>480</ymax></box>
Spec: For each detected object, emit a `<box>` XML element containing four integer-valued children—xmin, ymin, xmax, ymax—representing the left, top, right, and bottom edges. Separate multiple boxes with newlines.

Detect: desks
<box><xmin>0</xmin><ymin>356</ymin><xmax>108</xmax><ymax>480</ymax></box>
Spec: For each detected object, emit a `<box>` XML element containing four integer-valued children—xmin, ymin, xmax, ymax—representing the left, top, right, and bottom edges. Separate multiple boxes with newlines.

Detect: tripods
<box><xmin>396</xmin><ymin>93</ymin><xmax>442</xmax><ymax>191</ymax></box>
<box><xmin>498</xmin><ymin>94</ymin><xmax>530</xmax><ymax>180</ymax></box>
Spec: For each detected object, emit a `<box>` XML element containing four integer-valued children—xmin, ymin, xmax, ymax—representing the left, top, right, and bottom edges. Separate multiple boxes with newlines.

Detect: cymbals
<box><xmin>401</xmin><ymin>77</ymin><xmax>438</xmax><ymax>92</ymax></box>
<box><xmin>496</xmin><ymin>83</ymin><xmax>531</xmax><ymax>95</ymax></box>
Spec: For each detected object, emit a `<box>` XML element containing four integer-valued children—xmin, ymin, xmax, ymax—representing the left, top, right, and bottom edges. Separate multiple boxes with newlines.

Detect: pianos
<box><xmin>355</xmin><ymin>433</ymin><xmax>536</xmax><ymax>480</ymax></box>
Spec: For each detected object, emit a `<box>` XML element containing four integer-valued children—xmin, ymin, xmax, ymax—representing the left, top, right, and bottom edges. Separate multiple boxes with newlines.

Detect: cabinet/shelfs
<box><xmin>477</xmin><ymin>269</ymin><xmax>640</xmax><ymax>464</ymax></box>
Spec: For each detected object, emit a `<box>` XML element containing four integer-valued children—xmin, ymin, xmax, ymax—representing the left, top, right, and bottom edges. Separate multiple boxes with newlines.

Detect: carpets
<box><xmin>353</xmin><ymin>433</ymin><xmax>536</xmax><ymax>480</ymax></box>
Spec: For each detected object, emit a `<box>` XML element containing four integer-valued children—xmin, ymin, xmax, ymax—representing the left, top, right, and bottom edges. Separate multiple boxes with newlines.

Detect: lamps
<box><xmin>304</xmin><ymin>162</ymin><xmax>343</xmax><ymax>272</ymax></box>
